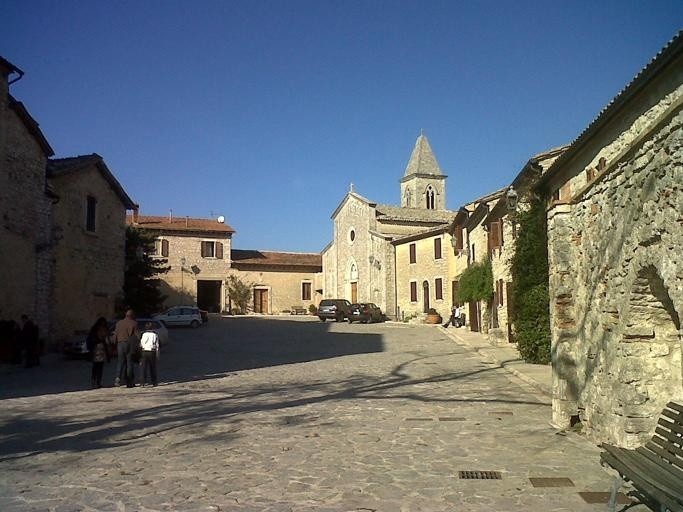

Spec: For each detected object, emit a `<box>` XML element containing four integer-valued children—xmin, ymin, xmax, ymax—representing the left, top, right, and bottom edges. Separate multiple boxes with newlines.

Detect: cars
<box><xmin>318</xmin><ymin>299</ymin><xmax>382</xmax><ymax>324</ymax></box>
<box><xmin>72</xmin><ymin>319</ymin><xmax>169</xmax><ymax>358</ymax></box>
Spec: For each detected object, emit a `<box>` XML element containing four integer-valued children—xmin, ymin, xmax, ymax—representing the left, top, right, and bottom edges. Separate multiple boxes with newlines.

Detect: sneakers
<box><xmin>89</xmin><ymin>375</ymin><xmax>160</xmax><ymax>388</ymax></box>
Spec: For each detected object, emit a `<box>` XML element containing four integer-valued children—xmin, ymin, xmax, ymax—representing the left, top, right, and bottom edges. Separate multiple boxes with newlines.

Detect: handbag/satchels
<box><xmin>93</xmin><ymin>342</ymin><xmax>106</xmax><ymax>363</ymax></box>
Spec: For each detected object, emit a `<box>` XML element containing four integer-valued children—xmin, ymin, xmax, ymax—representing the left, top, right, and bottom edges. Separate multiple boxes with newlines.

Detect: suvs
<box><xmin>152</xmin><ymin>306</ymin><xmax>209</xmax><ymax>328</ymax></box>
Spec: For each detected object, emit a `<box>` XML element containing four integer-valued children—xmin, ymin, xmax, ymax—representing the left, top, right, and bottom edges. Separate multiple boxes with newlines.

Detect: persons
<box><xmin>453</xmin><ymin>303</ymin><xmax>463</xmax><ymax>328</ymax></box>
<box><xmin>17</xmin><ymin>313</ymin><xmax>39</xmax><ymax>370</ymax></box>
<box><xmin>134</xmin><ymin>320</ymin><xmax>161</xmax><ymax>388</ymax></box>
<box><xmin>107</xmin><ymin>309</ymin><xmax>142</xmax><ymax>389</ymax></box>
<box><xmin>82</xmin><ymin>316</ymin><xmax>113</xmax><ymax>389</ymax></box>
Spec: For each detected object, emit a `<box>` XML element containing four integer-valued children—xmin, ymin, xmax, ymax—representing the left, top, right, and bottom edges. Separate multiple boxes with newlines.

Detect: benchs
<box><xmin>599</xmin><ymin>402</ymin><xmax>683</xmax><ymax>512</ymax></box>
